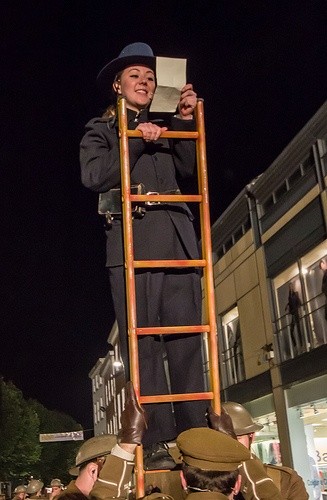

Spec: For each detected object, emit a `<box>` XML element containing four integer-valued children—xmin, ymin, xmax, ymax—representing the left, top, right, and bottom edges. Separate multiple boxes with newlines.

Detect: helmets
<box><xmin>50</xmin><ymin>479</ymin><xmax>64</xmax><ymax>487</ymax></box>
<box><xmin>75</xmin><ymin>434</ymin><xmax>117</xmax><ymax>466</ymax></box>
<box><xmin>27</xmin><ymin>480</ymin><xmax>44</xmax><ymax>493</ymax></box>
<box><xmin>222</xmin><ymin>401</ymin><xmax>264</xmax><ymax>435</ymax></box>
<box><xmin>13</xmin><ymin>485</ymin><xmax>27</xmax><ymax>493</ymax></box>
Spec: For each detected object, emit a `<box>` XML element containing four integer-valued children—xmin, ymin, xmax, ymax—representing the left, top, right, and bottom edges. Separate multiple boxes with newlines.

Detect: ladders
<box><xmin>117</xmin><ymin>99</ymin><xmax>221</xmax><ymax>500</ymax></box>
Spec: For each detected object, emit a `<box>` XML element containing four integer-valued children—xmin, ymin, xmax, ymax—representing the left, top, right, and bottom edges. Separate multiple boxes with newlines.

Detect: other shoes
<box><xmin>144</xmin><ymin>440</ymin><xmax>176</xmax><ymax>469</ymax></box>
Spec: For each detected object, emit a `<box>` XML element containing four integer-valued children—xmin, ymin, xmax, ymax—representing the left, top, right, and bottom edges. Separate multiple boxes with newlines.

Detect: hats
<box><xmin>69</xmin><ymin>466</ymin><xmax>80</xmax><ymax>476</ymax></box>
<box><xmin>96</xmin><ymin>42</ymin><xmax>156</xmax><ymax>82</ymax></box>
<box><xmin>176</xmin><ymin>427</ymin><xmax>251</xmax><ymax>471</ymax></box>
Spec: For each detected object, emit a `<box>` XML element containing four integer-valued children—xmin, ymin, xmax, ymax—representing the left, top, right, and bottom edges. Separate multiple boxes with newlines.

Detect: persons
<box><xmin>319</xmin><ymin>259</ymin><xmax>327</xmax><ymax>320</ymax></box>
<box><xmin>88</xmin><ymin>381</ymin><xmax>283</xmax><ymax>500</ymax></box>
<box><xmin>80</xmin><ymin>43</ymin><xmax>210</xmax><ymax>468</ymax></box>
<box><xmin>27</xmin><ymin>480</ymin><xmax>48</xmax><ymax>500</ymax></box>
<box><xmin>11</xmin><ymin>485</ymin><xmax>28</xmax><ymax>500</ymax></box>
<box><xmin>49</xmin><ymin>479</ymin><xmax>64</xmax><ymax>500</ymax></box>
<box><xmin>220</xmin><ymin>401</ymin><xmax>309</xmax><ymax>500</ymax></box>
<box><xmin>284</xmin><ymin>283</ymin><xmax>303</xmax><ymax>352</ymax></box>
<box><xmin>54</xmin><ymin>433</ymin><xmax>118</xmax><ymax>500</ymax></box>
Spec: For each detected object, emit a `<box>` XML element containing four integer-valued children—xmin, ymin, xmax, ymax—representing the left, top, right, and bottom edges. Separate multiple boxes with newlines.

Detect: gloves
<box><xmin>117</xmin><ymin>382</ymin><xmax>148</xmax><ymax>446</ymax></box>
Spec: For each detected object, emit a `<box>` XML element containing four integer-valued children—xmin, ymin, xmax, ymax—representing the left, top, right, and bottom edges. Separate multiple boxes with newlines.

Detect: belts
<box><xmin>146</xmin><ymin>189</ymin><xmax>195</xmax><ymax>220</ymax></box>
